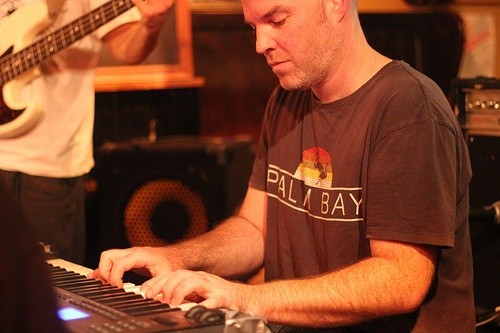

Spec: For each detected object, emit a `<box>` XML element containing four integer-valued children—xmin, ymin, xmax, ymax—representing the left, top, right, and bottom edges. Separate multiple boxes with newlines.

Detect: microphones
<box><xmin>469</xmin><ymin>200</ymin><xmax>500</xmax><ymax>224</ymax></box>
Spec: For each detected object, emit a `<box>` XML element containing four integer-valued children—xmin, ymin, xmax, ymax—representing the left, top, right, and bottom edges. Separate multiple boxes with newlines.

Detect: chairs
<box><xmin>358</xmin><ymin>11</ymin><xmax>465</xmax><ymax>131</ymax></box>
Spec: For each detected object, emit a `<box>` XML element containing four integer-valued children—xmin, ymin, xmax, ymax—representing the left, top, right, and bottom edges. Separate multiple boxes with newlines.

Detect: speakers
<box><xmin>86</xmin><ymin>137</ymin><xmax>230</xmax><ymax>285</ymax></box>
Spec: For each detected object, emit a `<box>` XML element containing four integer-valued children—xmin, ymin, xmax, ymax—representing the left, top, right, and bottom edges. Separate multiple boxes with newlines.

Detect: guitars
<box><xmin>0</xmin><ymin>0</ymin><xmax>135</xmax><ymax>139</ymax></box>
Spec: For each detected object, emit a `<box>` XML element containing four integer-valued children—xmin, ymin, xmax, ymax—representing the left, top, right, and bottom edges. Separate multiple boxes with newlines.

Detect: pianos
<box><xmin>1</xmin><ymin>256</ymin><xmax>270</xmax><ymax>332</ymax></box>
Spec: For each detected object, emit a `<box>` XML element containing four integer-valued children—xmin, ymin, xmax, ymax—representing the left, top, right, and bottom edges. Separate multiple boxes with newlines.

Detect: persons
<box><xmin>0</xmin><ymin>0</ymin><xmax>178</xmax><ymax>264</ymax></box>
<box><xmin>86</xmin><ymin>0</ymin><xmax>478</xmax><ymax>333</ymax></box>
